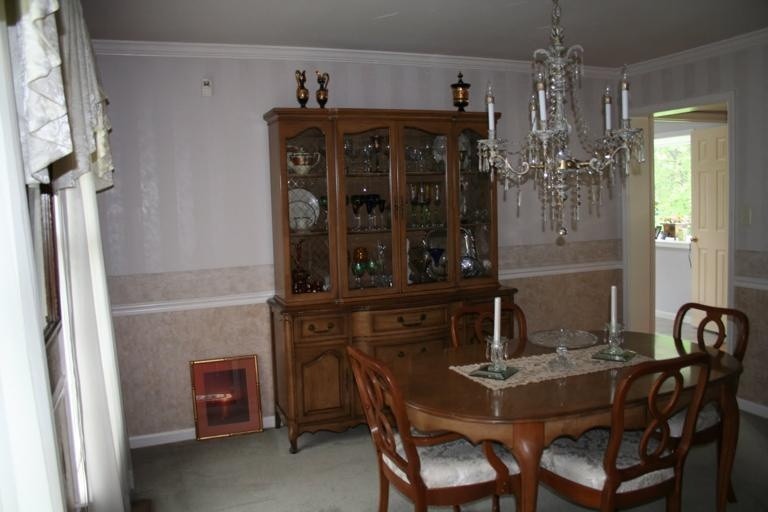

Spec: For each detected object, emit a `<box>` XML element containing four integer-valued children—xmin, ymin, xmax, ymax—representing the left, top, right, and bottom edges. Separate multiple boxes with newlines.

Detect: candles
<box><xmin>609</xmin><ymin>284</ymin><xmax>618</xmax><ymax>334</ymax></box>
<box><xmin>492</xmin><ymin>296</ymin><xmax>502</xmax><ymax>347</ymax></box>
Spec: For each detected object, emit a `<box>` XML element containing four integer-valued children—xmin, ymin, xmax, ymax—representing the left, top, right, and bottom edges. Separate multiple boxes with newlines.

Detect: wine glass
<box><xmin>321</xmin><ymin>136</ymin><xmax>473</xmax><ymax>232</ymax></box>
<box><xmin>353</xmin><ymin>250</ymin><xmax>445</xmax><ymax>288</ymax></box>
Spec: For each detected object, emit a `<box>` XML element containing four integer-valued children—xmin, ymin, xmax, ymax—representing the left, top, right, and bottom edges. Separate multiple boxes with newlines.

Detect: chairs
<box><xmin>648</xmin><ymin>302</ymin><xmax>750</xmax><ymax>503</ymax></box>
<box><xmin>446</xmin><ymin>299</ymin><xmax>528</xmax><ymax>348</ymax></box>
<box><xmin>342</xmin><ymin>345</ymin><xmax>525</xmax><ymax>512</ymax></box>
<box><xmin>538</xmin><ymin>352</ymin><xmax>711</xmax><ymax>512</ymax></box>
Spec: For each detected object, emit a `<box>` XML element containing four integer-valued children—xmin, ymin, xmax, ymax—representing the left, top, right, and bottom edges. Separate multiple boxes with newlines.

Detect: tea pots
<box><xmin>287</xmin><ymin>147</ymin><xmax>321</xmax><ymax>175</ymax></box>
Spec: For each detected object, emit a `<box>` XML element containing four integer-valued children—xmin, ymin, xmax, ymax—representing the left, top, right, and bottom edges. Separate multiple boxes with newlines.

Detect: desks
<box><xmin>369</xmin><ymin>330</ymin><xmax>742</xmax><ymax>512</ymax></box>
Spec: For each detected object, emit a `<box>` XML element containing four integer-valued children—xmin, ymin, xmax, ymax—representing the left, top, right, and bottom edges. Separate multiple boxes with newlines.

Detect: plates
<box><xmin>527</xmin><ymin>328</ymin><xmax>598</xmax><ymax>349</ymax></box>
<box><xmin>287</xmin><ymin>188</ymin><xmax>319</xmax><ymax>231</ymax></box>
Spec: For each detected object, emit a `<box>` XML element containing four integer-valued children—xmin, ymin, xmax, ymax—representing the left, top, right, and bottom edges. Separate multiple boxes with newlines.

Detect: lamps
<box><xmin>477</xmin><ymin>0</ymin><xmax>648</xmax><ymax>237</ymax></box>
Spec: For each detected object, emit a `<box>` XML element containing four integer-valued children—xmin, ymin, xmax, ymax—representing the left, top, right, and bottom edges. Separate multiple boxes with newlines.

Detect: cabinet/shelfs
<box><xmin>265</xmin><ymin>297</ymin><xmax>350</xmax><ymax>453</ymax></box>
<box><xmin>343</xmin><ymin>299</ymin><xmax>453</xmax><ymax>431</ymax></box>
<box><xmin>261</xmin><ymin>109</ymin><xmax>501</xmax><ymax>308</ymax></box>
<box><xmin>447</xmin><ymin>288</ymin><xmax>517</xmax><ymax>351</ymax></box>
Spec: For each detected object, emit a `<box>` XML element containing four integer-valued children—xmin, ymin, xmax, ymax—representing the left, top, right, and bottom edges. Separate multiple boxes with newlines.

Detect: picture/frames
<box><xmin>654</xmin><ymin>226</ymin><xmax>661</xmax><ymax>239</ymax></box>
<box><xmin>188</xmin><ymin>354</ymin><xmax>265</xmax><ymax>442</ymax></box>
